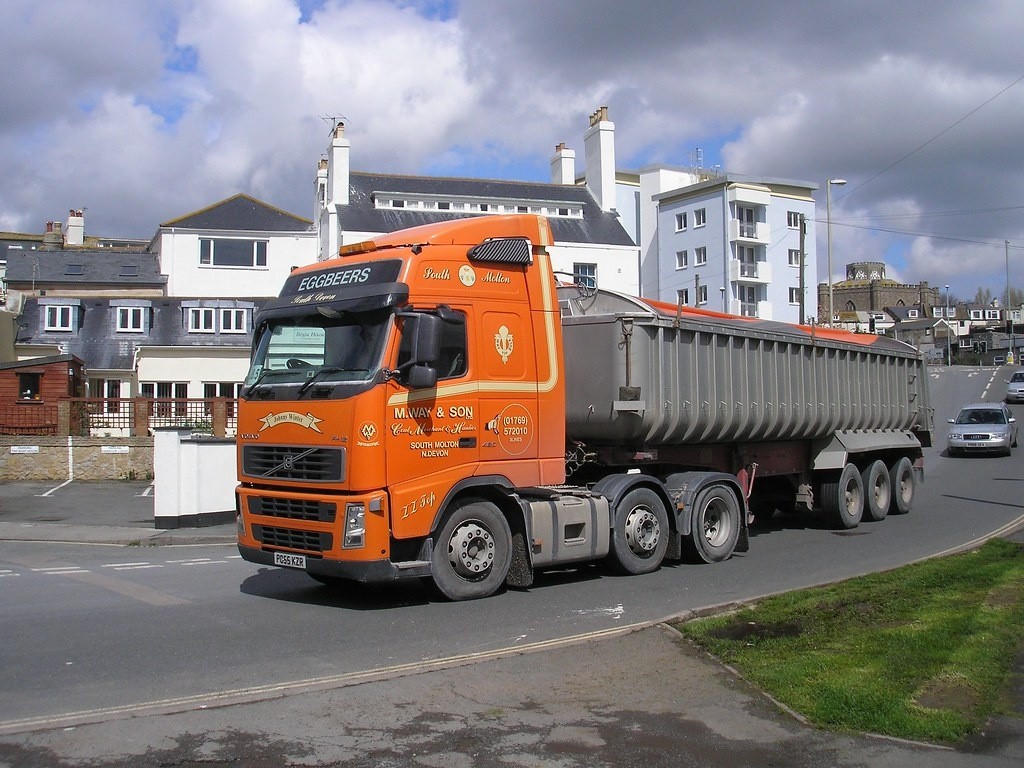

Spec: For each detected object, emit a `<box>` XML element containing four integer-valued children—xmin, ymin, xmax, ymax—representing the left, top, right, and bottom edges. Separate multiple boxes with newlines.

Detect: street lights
<box><xmin>828</xmin><ymin>179</ymin><xmax>846</xmax><ymax>329</ymax></box>
<box><xmin>945</xmin><ymin>285</ymin><xmax>951</xmax><ymax>366</ymax></box>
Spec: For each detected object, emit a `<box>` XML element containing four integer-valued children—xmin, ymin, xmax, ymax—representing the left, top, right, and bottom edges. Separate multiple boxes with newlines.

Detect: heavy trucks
<box><xmin>234</xmin><ymin>215</ymin><xmax>931</xmax><ymax>602</ymax></box>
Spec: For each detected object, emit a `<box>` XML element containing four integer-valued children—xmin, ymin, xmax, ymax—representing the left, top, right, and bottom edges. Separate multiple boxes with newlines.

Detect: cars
<box><xmin>1005</xmin><ymin>372</ymin><xmax>1024</xmax><ymax>403</ymax></box>
<box><xmin>947</xmin><ymin>403</ymin><xmax>1018</xmax><ymax>457</ymax></box>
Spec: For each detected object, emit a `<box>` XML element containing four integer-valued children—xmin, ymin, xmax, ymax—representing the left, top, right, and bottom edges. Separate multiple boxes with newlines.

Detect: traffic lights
<box><xmin>973</xmin><ymin>341</ymin><xmax>979</xmax><ymax>354</ymax></box>
<box><xmin>980</xmin><ymin>341</ymin><xmax>987</xmax><ymax>353</ymax></box>
<box><xmin>1005</xmin><ymin>320</ymin><xmax>1013</xmax><ymax>335</ymax></box>
